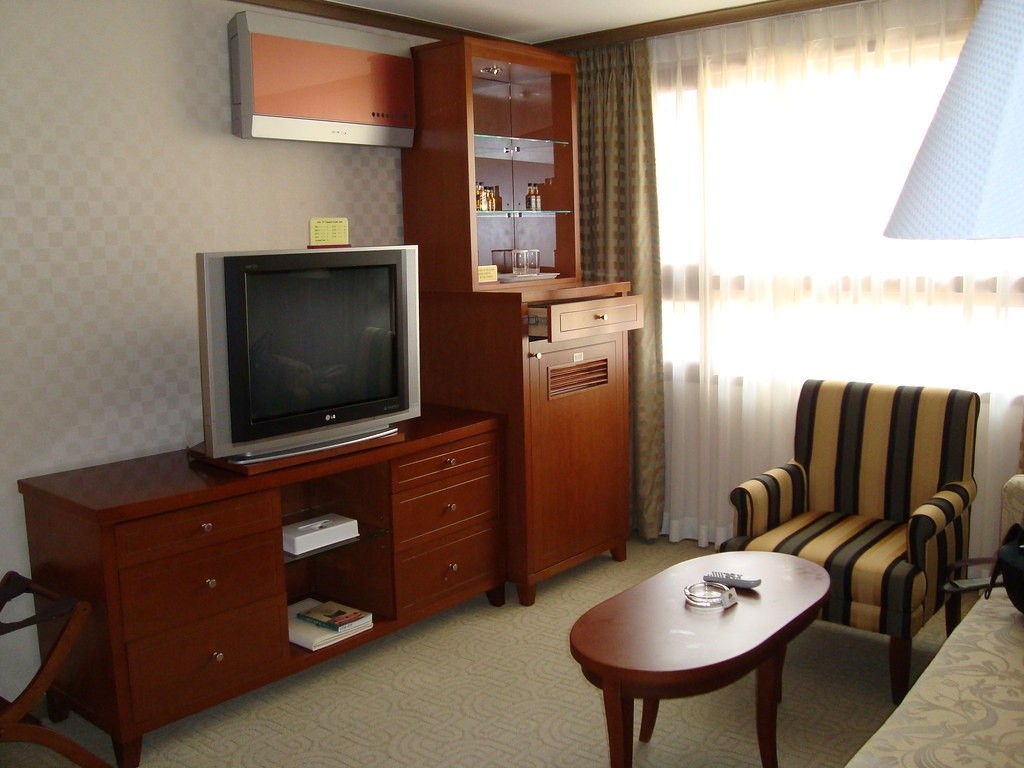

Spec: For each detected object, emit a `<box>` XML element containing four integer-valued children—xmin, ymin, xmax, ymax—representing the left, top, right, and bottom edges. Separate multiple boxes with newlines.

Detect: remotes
<box><xmin>704</xmin><ymin>570</ymin><xmax>762</xmax><ymax>587</ymax></box>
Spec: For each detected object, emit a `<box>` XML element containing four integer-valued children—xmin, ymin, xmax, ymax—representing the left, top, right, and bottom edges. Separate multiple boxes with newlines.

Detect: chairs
<box><xmin>717</xmin><ymin>376</ymin><xmax>981</xmax><ymax>707</ymax></box>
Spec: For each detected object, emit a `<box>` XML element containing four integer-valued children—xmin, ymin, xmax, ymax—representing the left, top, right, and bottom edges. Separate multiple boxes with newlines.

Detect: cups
<box><xmin>527</xmin><ymin>250</ymin><xmax>541</xmax><ymax>274</ymax></box>
<box><xmin>504</xmin><ymin>250</ymin><xmax>513</xmax><ymax>273</ymax></box>
<box><xmin>491</xmin><ymin>250</ymin><xmax>504</xmax><ymax>273</ymax></box>
<box><xmin>511</xmin><ymin>250</ymin><xmax>528</xmax><ymax>275</ymax></box>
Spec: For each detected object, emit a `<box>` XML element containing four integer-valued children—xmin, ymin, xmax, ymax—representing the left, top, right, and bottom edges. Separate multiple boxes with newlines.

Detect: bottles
<box><xmin>487</xmin><ymin>187</ymin><xmax>492</xmax><ymax>201</ymax></box>
<box><xmin>476</xmin><ymin>182</ymin><xmax>480</xmax><ymax>210</ymax></box>
<box><xmin>533</xmin><ymin>183</ymin><xmax>541</xmax><ymax>210</ymax></box>
<box><xmin>478</xmin><ymin>182</ymin><xmax>484</xmax><ymax>210</ymax></box>
<box><xmin>526</xmin><ymin>182</ymin><xmax>534</xmax><ymax>210</ymax></box>
<box><xmin>488</xmin><ymin>187</ymin><xmax>495</xmax><ymax>211</ymax></box>
<box><xmin>482</xmin><ymin>187</ymin><xmax>488</xmax><ymax>210</ymax></box>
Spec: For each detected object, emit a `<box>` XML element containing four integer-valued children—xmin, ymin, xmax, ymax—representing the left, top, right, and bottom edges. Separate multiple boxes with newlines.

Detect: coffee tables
<box><xmin>570</xmin><ymin>550</ymin><xmax>832</xmax><ymax>768</ymax></box>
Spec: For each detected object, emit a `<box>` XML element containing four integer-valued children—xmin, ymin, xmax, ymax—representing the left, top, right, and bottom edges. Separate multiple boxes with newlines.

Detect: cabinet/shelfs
<box><xmin>399</xmin><ymin>34</ymin><xmax>649</xmax><ymax>607</ymax></box>
<box><xmin>16</xmin><ymin>401</ymin><xmax>509</xmax><ymax>768</ymax></box>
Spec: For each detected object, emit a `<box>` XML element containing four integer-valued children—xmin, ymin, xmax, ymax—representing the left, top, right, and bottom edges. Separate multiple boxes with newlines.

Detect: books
<box><xmin>288</xmin><ymin>597</ymin><xmax>374</xmax><ymax>652</ymax></box>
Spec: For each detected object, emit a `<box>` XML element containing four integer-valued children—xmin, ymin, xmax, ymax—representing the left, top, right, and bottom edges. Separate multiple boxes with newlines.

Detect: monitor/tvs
<box><xmin>195</xmin><ymin>244</ymin><xmax>421</xmax><ymax>464</ymax></box>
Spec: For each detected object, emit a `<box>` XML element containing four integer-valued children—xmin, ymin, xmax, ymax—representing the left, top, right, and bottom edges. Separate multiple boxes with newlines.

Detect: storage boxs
<box><xmin>283</xmin><ymin>512</ymin><xmax>361</xmax><ymax>556</ymax></box>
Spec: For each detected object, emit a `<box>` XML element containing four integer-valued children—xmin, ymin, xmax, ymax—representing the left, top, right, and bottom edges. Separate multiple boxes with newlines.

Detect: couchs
<box><xmin>843</xmin><ymin>473</ymin><xmax>1024</xmax><ymax>768</ymax></box>
